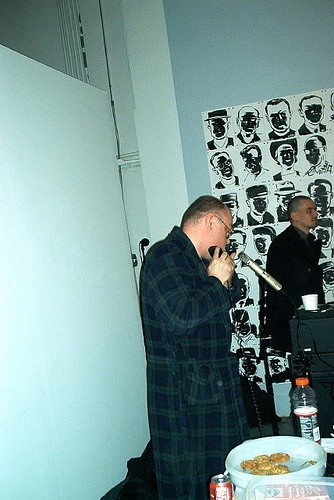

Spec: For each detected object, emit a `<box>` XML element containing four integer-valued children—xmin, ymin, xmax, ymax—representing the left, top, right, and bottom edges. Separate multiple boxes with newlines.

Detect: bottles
<box><xmin>293</xmin><ymin>378</ymin><xmax>321</xmax><ymax>445</ymax></box>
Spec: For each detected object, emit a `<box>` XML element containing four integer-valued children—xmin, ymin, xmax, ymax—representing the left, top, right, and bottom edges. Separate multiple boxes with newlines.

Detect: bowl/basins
<box><xmin>224</xmin><ymin>436</ymin><xmax>327</xmax><ymax>488</ymax></box>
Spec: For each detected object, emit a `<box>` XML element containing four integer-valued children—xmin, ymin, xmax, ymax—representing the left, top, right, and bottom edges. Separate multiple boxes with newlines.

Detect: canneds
<box><xmin>210</xmin><ymin>474</ymin><xmax>233</xmax><ymax>500</ymax></box>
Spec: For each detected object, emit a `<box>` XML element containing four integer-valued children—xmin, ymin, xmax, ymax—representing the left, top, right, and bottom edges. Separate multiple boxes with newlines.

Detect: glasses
<box><xmin>207</xmin><ymin>212</ymin><xmax>234</xmax><ymax>239</ymax></box>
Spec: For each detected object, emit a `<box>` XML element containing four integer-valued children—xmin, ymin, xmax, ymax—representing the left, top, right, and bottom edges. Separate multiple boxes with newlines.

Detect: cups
<box><xmin>302</xmin><ymin>294</ymin><xmax>318</xmax><ymax>310</ymax></box>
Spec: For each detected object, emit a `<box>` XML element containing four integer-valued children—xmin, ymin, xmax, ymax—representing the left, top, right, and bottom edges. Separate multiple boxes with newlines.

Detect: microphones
<box><xmin>238</xmin><ymin>252</ymin><xmax>290</xmax><ymax>299</ymax></box>
<box><xmin>209</xmin><ymin>246</ymin><xmax>241</xmax><ymax>290</ymax></box>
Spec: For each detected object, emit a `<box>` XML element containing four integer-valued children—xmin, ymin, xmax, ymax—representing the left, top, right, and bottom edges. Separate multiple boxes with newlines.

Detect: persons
<box><xmin>139</xmin><ymin>196</ymin><xmax>252</xmax><ymax>500</ymax></box>
<box><xmin>264</xmin><ymin>196</ymin><xmax>326</xmax><ymax>353</ymax></box>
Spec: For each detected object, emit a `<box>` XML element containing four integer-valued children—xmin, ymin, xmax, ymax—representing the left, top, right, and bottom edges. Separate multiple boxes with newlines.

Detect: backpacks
<box><xmin>239</xmin><ymin>374</ymin><xmax>283</xmax><ymax>427</ymax></box>
